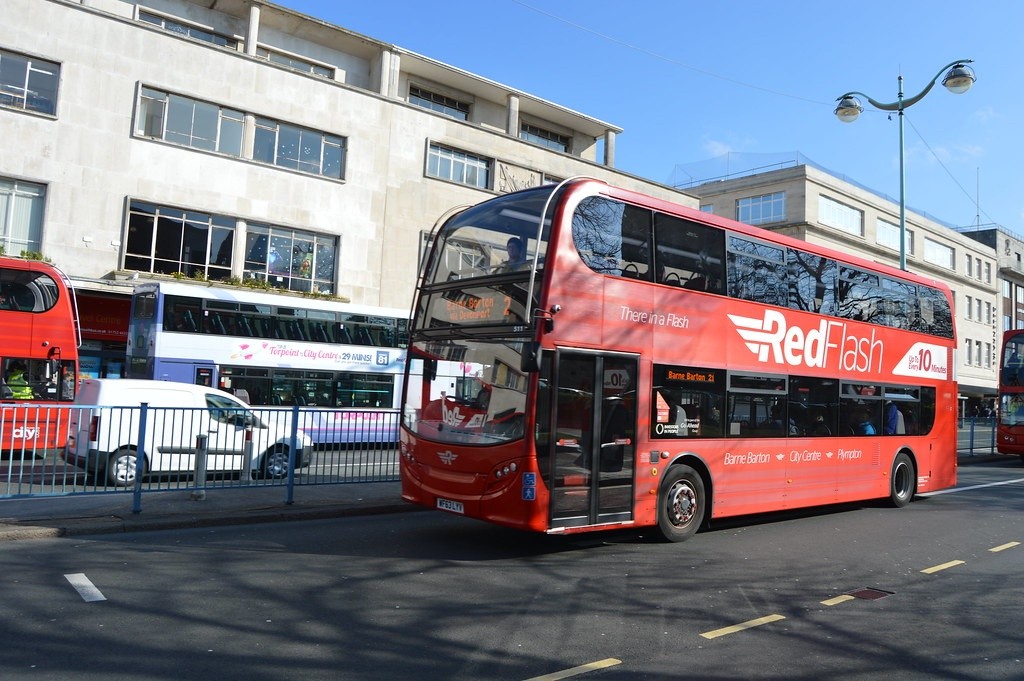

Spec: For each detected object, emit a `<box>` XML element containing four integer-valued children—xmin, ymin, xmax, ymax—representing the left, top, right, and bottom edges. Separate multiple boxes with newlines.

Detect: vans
<box><xmin>62</xmin><ymin>378</ymin><xmax>315</xmax><ymax>488</ymax></box>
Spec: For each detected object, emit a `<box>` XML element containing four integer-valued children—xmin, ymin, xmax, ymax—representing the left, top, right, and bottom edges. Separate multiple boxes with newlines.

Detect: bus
<box><xmin>0</xmin><ymin>257</ymin><xmax>85</xmax><ymax>453</ymax></box>
<box><xmin>125</xmin><ymin>282</ymin><xmax>491</xmax><ymax>445</ymax></box>
<box><xmin>997</xmin><ymin>330</ymin><xmax>1024</xmax><ymax>457</ymax></box>
<box><xmin>398</xmin><ymin>176</ymin><xmax>958</xmax><ymax>540</ymax></box>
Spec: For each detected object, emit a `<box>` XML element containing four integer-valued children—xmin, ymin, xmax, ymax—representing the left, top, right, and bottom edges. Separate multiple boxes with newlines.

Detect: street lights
<box><xmin>833</xmin><ymin>57</ymin><xmax>978</xmax><ymax>271</ymax></box>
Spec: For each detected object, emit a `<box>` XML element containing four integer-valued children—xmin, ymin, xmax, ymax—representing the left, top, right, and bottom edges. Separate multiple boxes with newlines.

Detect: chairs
<box><xmin>167</xmin><ymin>309</ymin><xmax>378</xmax><ymax>346</ymax></box>
<box><xmin>294</xmin><ymin>396</ymin><xmax>307</xmax><ymax>406</ymax></box>
<box><xmin>664</xmin><ymin>272</ymin><xmax>681</xmax><ymax>287</ymax></box>
<box><xmin>271</xmin><ymin>395</ymin><xmax>283</xmax><ymax>406</ymax></box>
<box><xmin>622</xmin><ymin>265</ymin><xmax>639</xmax><ymax>279</ymax></box>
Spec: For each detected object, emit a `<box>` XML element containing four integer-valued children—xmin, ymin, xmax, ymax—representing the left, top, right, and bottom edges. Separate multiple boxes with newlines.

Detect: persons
<box><xmin>478</xmin><ymin>367</ymin><xmax>504</xmax><ymax>410</ymax></box>
<box><xmin>770</xmin><ymin>405</ymin><xmax>795</xmax><ymax>434</ymax></box>
<box><xmin>884</xmin><ymin>399</ymin><xmax>898</xmax><ymax>435</ymax></box>
<box><xmin>1008</xmin><ymin>396</ymin><xmax>1019</xmax><ymax>417</ymax></box>
<box><xmin>7</xmin><ymin>361</ymin><xmax>41</xmax><ymax>399</ymax></box>
<box><xmin>63</xmin><ymin>373</ymin><xmax>71</xmax><ymax>395</ymax></box>
<box><xmin>503</xmin><ymin>237</ymin><xmax>525</xmax><ymax>267</ymax></box>
<box><xmin>855</xmin><ymin>411</ymin><xmax>876</xmax><ymax>435</ymax></box>
<box><xmin>974</xmin><ymin>406</ymin><xmax>979</xmax><ymax>422</ymax></box>
<box><xmin>984</xmin><ymin>406</ymin><xmax>996</xmax><ymax>424</ymax></box>
